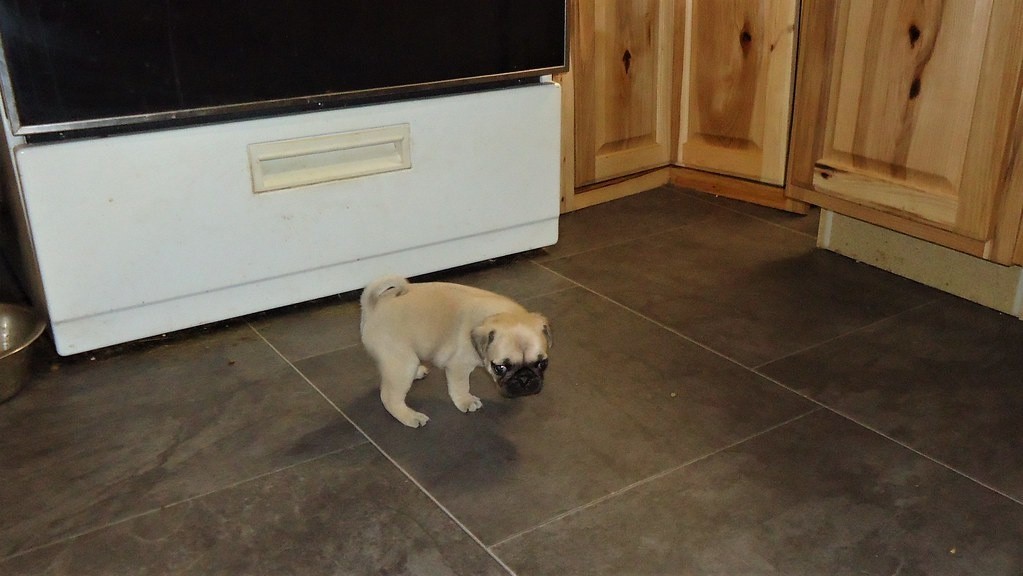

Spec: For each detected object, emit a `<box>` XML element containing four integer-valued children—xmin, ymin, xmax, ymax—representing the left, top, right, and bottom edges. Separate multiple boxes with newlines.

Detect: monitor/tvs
<box><xmin>1</xmin><ymin>0</ymin><xmax>569</xmax><ymax>135</ymax></box>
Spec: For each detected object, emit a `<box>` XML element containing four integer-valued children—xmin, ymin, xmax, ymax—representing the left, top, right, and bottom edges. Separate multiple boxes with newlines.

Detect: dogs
<box><xmin>357</xmin><ymin>272</ymin><xmax>553</xmax><ymax>428</ymax></box>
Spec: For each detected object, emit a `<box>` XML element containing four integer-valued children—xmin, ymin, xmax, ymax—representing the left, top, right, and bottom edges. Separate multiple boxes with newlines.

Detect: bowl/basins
<box><xmin>0</xmin><ymin>303</ymin><xmax>48</xmax><ymax>406</ymax></box>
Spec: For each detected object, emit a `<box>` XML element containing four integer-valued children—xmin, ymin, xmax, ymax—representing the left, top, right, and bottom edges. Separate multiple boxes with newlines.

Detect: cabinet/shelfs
<box><xmin>556</xmin><ymin>1</ymin><xmax>1022</xmax><ymax>318</ymax></box>
<box><xmin>0</xmin><ymin>0</ymin><xmax>566</xmax><ymax>358</ymax></box>
